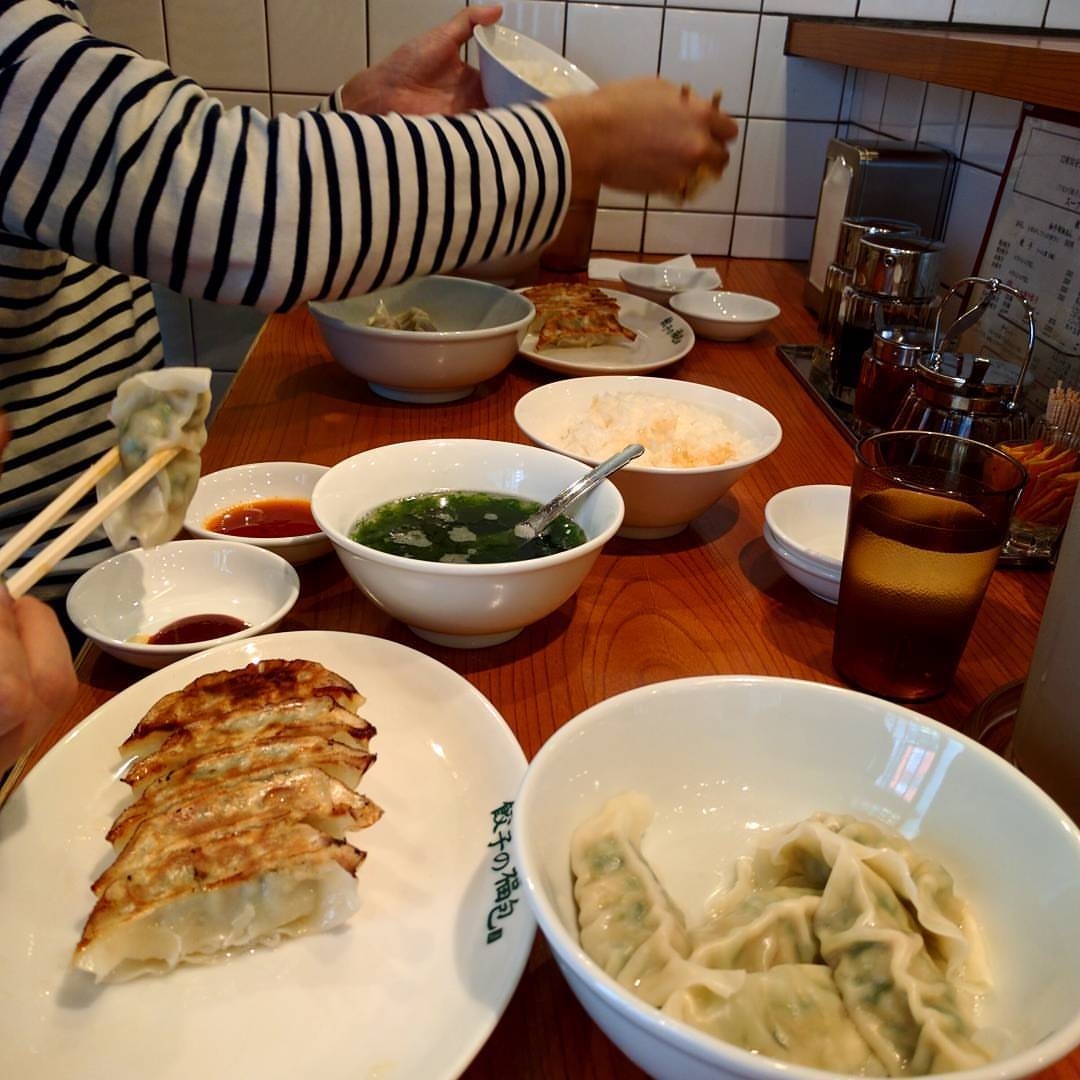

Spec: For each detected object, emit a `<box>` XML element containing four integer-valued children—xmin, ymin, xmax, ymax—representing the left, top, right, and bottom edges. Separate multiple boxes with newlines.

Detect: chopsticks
<box><xmin>1</xmin><ymin>445</ymin><xmax>186</xmax><ymax>599</ymax></box>
<box><xmin>678</xmin><ymin>81</ymin><xmax>727</xmax><ymax>115</ymax></box>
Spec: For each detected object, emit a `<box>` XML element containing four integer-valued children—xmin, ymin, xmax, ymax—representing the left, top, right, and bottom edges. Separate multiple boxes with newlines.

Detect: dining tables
<box><xmin>1</xmin><ymin>252</ymin><xmax>1079</xmax><ymax>1080</ymax></box>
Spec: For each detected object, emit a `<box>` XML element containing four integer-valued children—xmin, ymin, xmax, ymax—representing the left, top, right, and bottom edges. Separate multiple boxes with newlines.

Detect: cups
<box><xmin>811</xmin><ymin>211</ymin><xmax>948</xmax><ymax>433</ymax></box>
<box><xmin>1006</xmin><ymin>418</ymin><xmax>1080</xmax><ymax>566</ymax></box>
<box><xmin>828</xmin><ymin>426</ymin><xmax>1028</xmax><ymax>703</ymax></box>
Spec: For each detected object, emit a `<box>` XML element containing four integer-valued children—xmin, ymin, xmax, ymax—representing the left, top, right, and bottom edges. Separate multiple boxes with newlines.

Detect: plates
<box><xmin>1</xmin><ymin>630</ymin><xmax>529</xmax><ymax>1080</ymax></box>
<box><xmin>509</xmin><ymin>284</ymin><xmax>696</xmax><ymax>377</ymax></box>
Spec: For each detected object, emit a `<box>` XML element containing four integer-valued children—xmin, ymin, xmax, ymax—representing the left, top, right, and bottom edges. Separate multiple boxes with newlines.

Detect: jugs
<box><xmin>865</xmin><ymin>275</ymin><xmax>1038</xmax><ymax>480</ymax></box>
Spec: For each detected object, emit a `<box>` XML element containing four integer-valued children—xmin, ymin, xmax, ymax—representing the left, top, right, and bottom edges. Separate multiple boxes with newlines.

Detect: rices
<box><xmin>551</xmin><ymin>383</ymin><xmax>747</xmax><ymax>473</ymax></box>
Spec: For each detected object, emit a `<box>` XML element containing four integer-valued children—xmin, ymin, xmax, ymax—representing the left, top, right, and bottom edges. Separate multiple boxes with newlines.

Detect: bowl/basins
<box><xmin>310</xmin><ymin>438</ymin><xmax>625</xmax><ymax>650</ymax></box>
<box><xmin>308</xmin><ymin>279</ymin><xmax>535</xmax><ymax>404</ymax></box>
<box><xmin>66</xmin><ymin>536</ymin><xmax>302</xmax><ymax>667</ymax></box>
<box><xmin>668</xmin><ymin>289</ymin><xmax>779</xmax><ymax>343</ymax></box>
<box><xmin>512</xmin><ymin>374</ymin><xmax>785</xmax><ymax>541</ymax></box>
<box><xmin>512</xmin><ymin>672</ymin><xmax>1080</xmax><ymax>1080</ymax></box>
<box><xmin>613</xmin><ymin>265</ymin><xmax>723</xmax><ymax>308</ymax></box>
<box><xmin>474</xmin><ymin>19</ymin><xmax>601</xmax><ymax>116</ymax></box>
<box><xmin>183</xmin><ymin>460</ymin><xmax>340</xmax><ymax>562</ymax></box>
<box><xmin>760</xmin><ymin>480</ymin><xmax>853</xmax><ymax>605</ymax></box>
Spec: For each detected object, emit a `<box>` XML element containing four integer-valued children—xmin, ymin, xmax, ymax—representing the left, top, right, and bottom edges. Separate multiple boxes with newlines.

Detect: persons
<box><xmin>0</xmin><ymin>585</ymin><xmax>83</xmax><ymax>782</ymax></box>
<box><xmin>1</xmin><ymin>0</ymin><xmax>742</xmax><ymax>656</ymax></box>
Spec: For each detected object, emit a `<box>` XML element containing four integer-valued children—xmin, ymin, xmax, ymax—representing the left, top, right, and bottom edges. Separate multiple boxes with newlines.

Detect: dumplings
<box><xmin>570</xmin><ymin>794</ymin><xmax>1010</xmax><ymax>1080</ymax></box>
<box><xmin>86</xmin><ymin>362</ymin><xmax>217</xmax><ymax>557</ymax></box>
<box><xmin>61</xmin><ymin>657</ymin><xmax>377</xmax><ymax>999</ymax></box>
<box><xmin>520</xmin><ymin>278</ymin><xmax>642</xmax><ymax>353</ymax></box>
